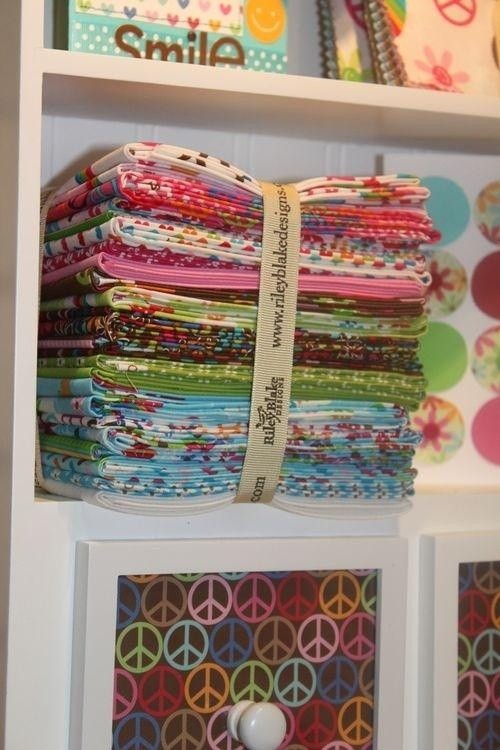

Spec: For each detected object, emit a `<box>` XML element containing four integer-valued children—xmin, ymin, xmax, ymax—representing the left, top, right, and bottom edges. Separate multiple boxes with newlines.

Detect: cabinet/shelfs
<box><xmin>1</xmin><ymin>0</ymin><xmax>500</xmax><ymax>750</ymax></box>
